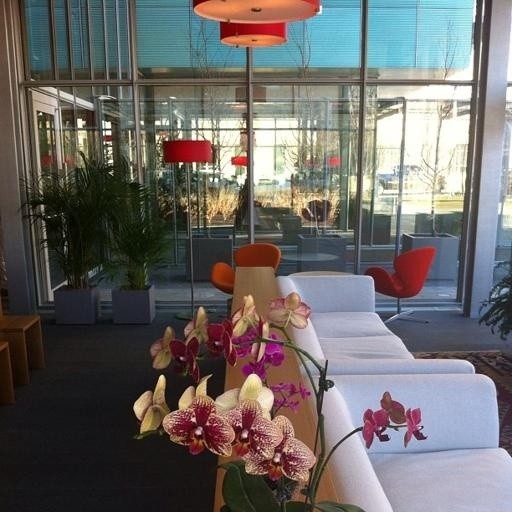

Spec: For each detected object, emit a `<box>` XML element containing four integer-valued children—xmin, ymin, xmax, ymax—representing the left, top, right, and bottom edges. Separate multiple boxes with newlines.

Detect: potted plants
<box><xmin>106</xmin><ymin>166</ymin><xmax>170</xmax><ymax>324</ymax></box>
<box><xmin>19</xmin><ymin>153</ymin><xmax>106</xmax><ymax>326</ymax></box>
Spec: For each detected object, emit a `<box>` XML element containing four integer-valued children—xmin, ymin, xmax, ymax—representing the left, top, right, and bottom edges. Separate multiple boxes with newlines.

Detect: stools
<box><xmin>0</xmin><ymin>337</ymin><xmax>16</xmax><ymax>408</ymax></box>
<box><xmin>0</xmin><ymin>313</ymin><xmax>47</xmax><ymax>386</ymax></box>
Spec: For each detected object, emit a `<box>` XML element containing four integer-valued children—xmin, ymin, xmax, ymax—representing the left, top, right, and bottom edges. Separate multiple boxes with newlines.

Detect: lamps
<box><xmin>192</xmin><ymin>1</ymin><xmax>324</xmax><ymax>24</ymax></box>
<box><xmin>161</xmin><ymin>140</ymin><xmax>214</xmax><ymax>324</ymax></box>
<box><xmin>231</xmin><ymin>158</ymin><xmax>247</xmax><ymax>186</ymax></box>
<box><xmin>217</xmin><ymin>22</ymin><xmax>287</xmax><ymax>46</ymax></box>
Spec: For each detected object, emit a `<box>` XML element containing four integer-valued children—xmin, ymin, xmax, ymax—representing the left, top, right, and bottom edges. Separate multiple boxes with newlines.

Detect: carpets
<box><xmin>410</xmin><ymin>347</ymin><xmax>512</xmax><ymax>457</ymax></box>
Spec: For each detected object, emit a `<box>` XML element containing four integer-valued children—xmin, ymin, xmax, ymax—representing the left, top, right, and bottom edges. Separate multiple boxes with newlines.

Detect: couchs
<box><xmin>213</xmin><ymin>266</ymin><xmax>512</xmax><ymax>512</ymax></box>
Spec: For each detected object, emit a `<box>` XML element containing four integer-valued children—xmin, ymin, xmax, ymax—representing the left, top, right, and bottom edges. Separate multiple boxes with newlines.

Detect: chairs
<box><xmin>366</xmin><ymin>246</ymin><xmax>436</xmax><ymax>326</ymax></box>
<box><xmin>302</xmin><ymin>199</ymin><xmax>332</xmax><ymax>236</ymax></box>
<box><xmin>210</xmin><ymin>242</ymin><xmax>281</xmax><ymax>298</ymax></box>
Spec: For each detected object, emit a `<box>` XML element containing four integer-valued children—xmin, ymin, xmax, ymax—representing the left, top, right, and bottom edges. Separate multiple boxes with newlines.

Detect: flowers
<box><xmin>127</xmin><ymin>288</ymin><xmax>429</xmax><ymax>510</ymax></box>
